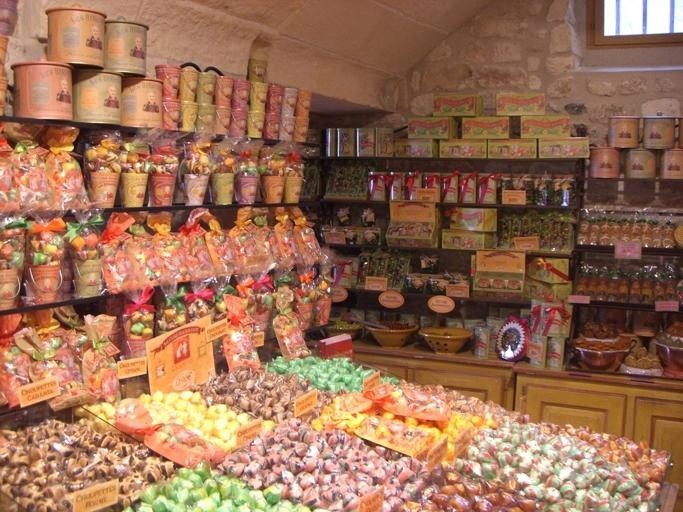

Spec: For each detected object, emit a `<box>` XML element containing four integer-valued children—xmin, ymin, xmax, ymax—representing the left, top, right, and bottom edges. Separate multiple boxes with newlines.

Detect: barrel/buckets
<box><xmin>7</xmin><ymin>7</ymin><xmax>163</xmax><ymax>127</ymax></box>
<box><xmin>589</xmin><ymin>111</ymin><xmax>683</xmax><ymax>179</ymax></box>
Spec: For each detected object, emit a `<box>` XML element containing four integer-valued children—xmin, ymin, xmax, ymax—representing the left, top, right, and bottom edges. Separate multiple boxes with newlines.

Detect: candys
<box><xmin>0</xmin><ymin>139</ymin><xmax>672</xmax><ymax>512</ymax></box>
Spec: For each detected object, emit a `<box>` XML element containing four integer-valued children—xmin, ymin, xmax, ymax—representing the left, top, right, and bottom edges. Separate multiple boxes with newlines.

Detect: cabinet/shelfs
<box><xmin>515</xmin><ymin>374</ymin><xmax>683</xmax><ymax>497</ymax></box>
<box><xmin>356</xmin><ymin>353</ymin><xmax>504</xmax><ymax>406</ymax></box>
<box><xmin>0</xmin><ymin>114</ymin><xmax>321</xmax><ymax>420</ymax></box>
<box><xmin>301</xmin><ymin>155</ymin><xmax>683</xmax><ymax>388</ymax></box>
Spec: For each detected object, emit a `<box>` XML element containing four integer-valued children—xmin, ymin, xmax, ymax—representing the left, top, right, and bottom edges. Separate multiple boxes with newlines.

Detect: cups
<box><xmin>86</xmin><ymin>170</ymin><xmax>303</xmax><ymax>205</ymax></box>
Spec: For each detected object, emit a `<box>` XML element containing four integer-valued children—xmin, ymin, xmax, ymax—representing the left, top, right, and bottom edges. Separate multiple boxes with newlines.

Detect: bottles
<box><xmin>10</xmin><ymin>10</ymin><xmax>164</xmax><ymax>130</ymax></box>
<box><xmin>589</xmin><ymin>112</ymin><xmax>683</xmax><ymax>181</ymax></box>
<box><xmin>496</xmin><ymin>171</ymin><xmax>576</xmax><ymax>207</ymax></box>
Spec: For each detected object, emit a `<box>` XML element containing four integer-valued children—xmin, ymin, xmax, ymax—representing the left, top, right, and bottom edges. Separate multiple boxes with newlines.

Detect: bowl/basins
<box><xmin>572</xmin><ymin>339</ymin><xmax>630</xmax><ymax>374</ymax></box>
<box><xmin>653</xmin><ymin>341</ymin><xmax>683</xmax><ymax>372</ymax></box>
<box><xmin>324</xmin><ymin>320</ymin><xmax>476</xmax><ymax>355</ymax></box>
